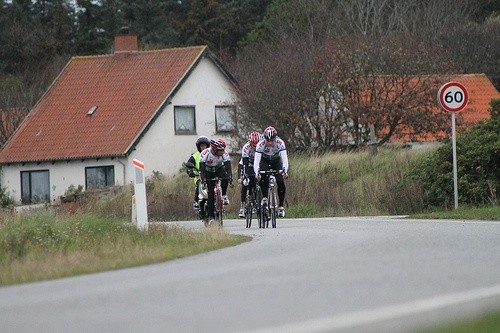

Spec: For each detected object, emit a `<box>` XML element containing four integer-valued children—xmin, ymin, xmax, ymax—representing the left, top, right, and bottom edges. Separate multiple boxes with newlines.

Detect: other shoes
<box><xmin>261</xmin><ymin>198</ymin><xmax>268</xmax><ymax>208</ymax></box>
<box><xmin>239</xmin><ymin>208</ymin><xmax>246</xmax><ymax>216</ymax></box>
<box><xmin>209</xmin><ymin>217</ymin><xmax>215</xmax><ymax>225</ymax></box>
<box><xmin>278</xmin><ymin>207</ymin><xmax>285</xmax><ymax>217</ymax></box>
<box><xmin>251</xmin><ymin>207</ymin><xmax>257</xmax><ymax>213</ymax></box>
<box><xmin>221</xmin><ymin>195</ymin><xmax>230</xmax><ymax>205</ymax></box>
<box><xmin>192</xmin><ymin>201</ymin><xmax>200</xmax><ymax>210</ymax></box>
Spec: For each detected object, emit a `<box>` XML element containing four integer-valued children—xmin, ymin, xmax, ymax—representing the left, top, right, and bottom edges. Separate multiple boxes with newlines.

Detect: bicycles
<box><xmin>238</xmin><ymin>170</ymin><xmax>269</xmax><ymax>229</ymax></box>
<box><xmin>200</xmin><ymin>176</ymin><xmax>234</xmax><ymax>231</ymax></box>
<box><xmin>257</xmin><ymin>167</ymin><xmax>288</xmax><ymax>228</ymax></box>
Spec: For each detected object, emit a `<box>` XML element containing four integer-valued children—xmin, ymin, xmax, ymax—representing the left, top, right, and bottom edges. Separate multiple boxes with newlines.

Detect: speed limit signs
<box><xmin>437</xmin><ymin>82</ymin><xmax>469</xmax><ymax>114</ymax></box>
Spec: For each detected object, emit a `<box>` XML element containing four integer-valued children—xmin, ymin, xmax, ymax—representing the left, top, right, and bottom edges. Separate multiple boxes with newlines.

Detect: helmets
<box><xmin>248</xmin><ymin>131</ymin><xmax>261</xmax><ymax>146</ymax></box>
<box><xmin>196</xmin><ymin>136</ymin><xmax>210</xmax><ymax>152</ymax></box>
<box><xmin>264</xmin><ymin>126</ymin><xmax>277</xmax><ymax>139</ymax></box>
<box><xmin>210</xmin><ymin>139</ymin><xmax>226</xmax><ymax>153</ymax></box>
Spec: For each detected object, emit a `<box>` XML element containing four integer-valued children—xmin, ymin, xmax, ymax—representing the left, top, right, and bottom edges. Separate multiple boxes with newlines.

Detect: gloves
<box><xmin>188</xmin><ymin>170</ymin><xmax>196</xmax><ymax>177</ymax></box>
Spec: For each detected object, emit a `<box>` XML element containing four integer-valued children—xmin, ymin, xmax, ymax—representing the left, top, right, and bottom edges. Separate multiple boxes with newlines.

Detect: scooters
<box><xmin>185</xmin><ymin>162</ymin><xmax>216</xmax><ymax>228</ymax></box>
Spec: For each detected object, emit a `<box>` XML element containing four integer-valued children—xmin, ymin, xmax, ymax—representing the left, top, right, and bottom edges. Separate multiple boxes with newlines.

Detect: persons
<box><xmin>253</xmin><ymin>127</ymin><xmax>288</xmax><ymax>217</ymax></box>
<box><xmin>238</xmin><ymin>131</ymin><xmax>270</xmax><ymax>221</ymax></box>
<box><xmin>199</xmin><ymin>138</ymin><xmax>234</xmax><ymax>225</ymax></box>
<box><xmin>186</xmin><ymin>136</ymin><xmax>210</xmax><ymax>209</ymax></box>
